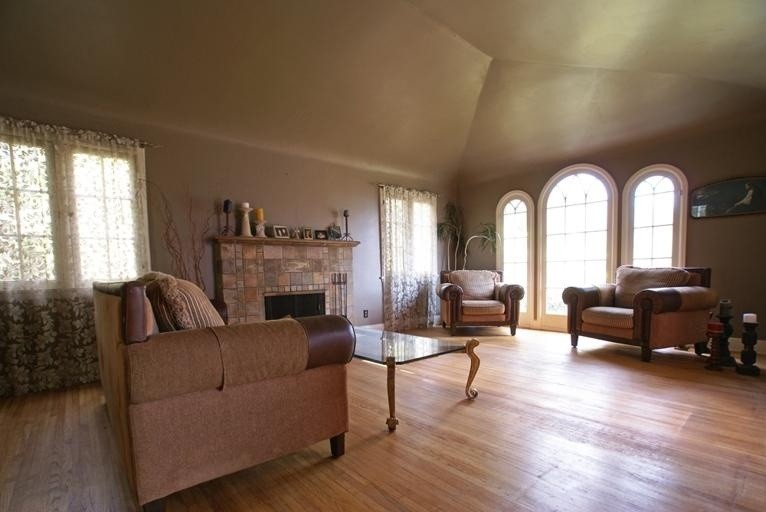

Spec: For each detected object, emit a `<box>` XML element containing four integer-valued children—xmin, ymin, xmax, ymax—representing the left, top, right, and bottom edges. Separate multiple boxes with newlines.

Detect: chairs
<box><xmin>436</xmin><ymin>271</ymin><xmax>524</xmax><ymax>336</ymax></box>
<box><xmin>562</xmin><ymin>265</ymin><xmax>719</xmax><ymax>362</ymax></box>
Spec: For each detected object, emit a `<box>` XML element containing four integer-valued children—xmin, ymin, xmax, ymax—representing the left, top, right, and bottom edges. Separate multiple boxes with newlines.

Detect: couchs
<box><xmin>93</xmin><ymin>282</ymin><xmax>356</xmax><ymax>512</ymax></box>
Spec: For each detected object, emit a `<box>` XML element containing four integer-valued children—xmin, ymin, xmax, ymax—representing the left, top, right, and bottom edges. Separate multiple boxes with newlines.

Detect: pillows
<box><xmin>449</xmin><ymin>270</ymin><xmax>498</xmax><ymax>300</ymax></box>
<box><xmin>137</xmin><ymin>272</ymin><xmax>226</xmax><ymax>331</ymax></box>
<box><xmin>614</xmin><ymin>265</ymin><xmax>690</xmax><ymax>308</ymax></box>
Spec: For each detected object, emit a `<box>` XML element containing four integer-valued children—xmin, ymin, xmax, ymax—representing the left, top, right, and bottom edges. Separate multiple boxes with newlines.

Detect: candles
<box><xmin>241</xmin><ymin>202</ymin><xmax>250</xmax><ymax>208</ymax></box>
<box><xmin>344</xmin><ymin>210</ymin><xmax>350</xmax><ymax>217</ymax></box>
<box><xmin>255</xmin><ymin>208</ymin><xmax>264</xmax><ymax>222</ymax></box>
<box><xmin>224</xmin><ymin>198</ymin><xmax>232</xmax><ymax>212</ymax></box>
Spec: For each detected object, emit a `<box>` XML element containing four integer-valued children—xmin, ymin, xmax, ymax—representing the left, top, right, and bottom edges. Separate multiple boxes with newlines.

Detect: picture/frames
<box><xmin>688</xmin><ymin>176</ymin><xmax>766</xmax><ymax>219</ymax></box>
<box><xmin>304</xmin><ymin>228</ymin><xmax>313</xmax><ymax>240</ymax></box>
<box><xmin>273</xmin><ymin>226</ymin><xmax>289</xmax><ymax>238</ymax></box>
<box><xmin>315</xmin><ymin>230</ymin><xmax>327</xmax><ymax>239</ymax></box>
